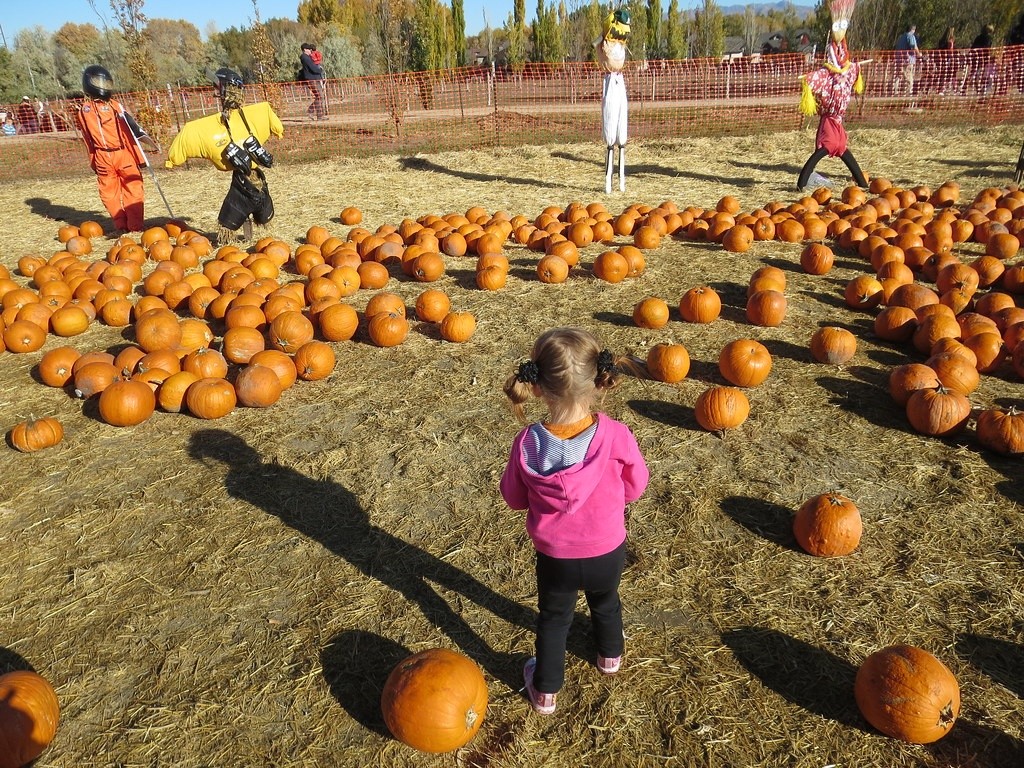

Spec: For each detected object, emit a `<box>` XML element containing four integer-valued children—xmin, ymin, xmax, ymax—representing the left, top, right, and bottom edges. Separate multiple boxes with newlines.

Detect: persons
<box><xmin>299</xmin><ymin>43</ymin><xmax>329</xmax><ymax>122</ymax></box>
<box><xmin>76</xmin><ymin>65</ymin><xmax>147</xmax><ymax>233</ymax></box>
<box><xmin>925</xmin><ymin>26</ymin><xmax>954</xmax><ymax>97</ymax></box>
<box><xmin>165</xmin><ymin>68</ymin><xmax>284</xmax><ymax>241</ymax></box>
<box><xmin>795</xmin><ymin>36</ymin><xmax>869</xmax><ymax>192</ymax></box>
<box><xmin>960</xmin><ymin>22</ymin><xmax>994</xmax><ymax>96</ymax></box>
<box><xmin>998</xmin><ymin>11</ymin><xmax>1024</xmax><ymax>95</ymax></box>
<box><xmin>893</xmin><ymin>24</ymin><xmax>922</xmax><ymax>95</ymax></box>
<box><xmin>500</xmin><ymin>327</ymin><xmax>649</xmax><ymax>714</ymax></box>
<box><xmin>0</xmin><ymin>95</ymin><xmax>45</xmax><ymax>137</ymax></box>
<box><xmin>598</xmin><ymin>10</ymin><xmax>632</xmax><ymax>193</ymax></box>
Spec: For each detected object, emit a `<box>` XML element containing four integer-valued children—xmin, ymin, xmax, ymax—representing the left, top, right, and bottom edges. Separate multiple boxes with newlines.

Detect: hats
<box><xmin>614</xmin><ymin>9</ymin><xmax>632</xmax><ymax>25</ymax></box>
<box><xmin>301</xmin><ymin>43</ymin><xmax>313</xmax><ymax>50</ymax></box>
<box><xmin>23</xmin><ymin>95</ymin><xmax>30</xmax><ymax>99</ymax></box>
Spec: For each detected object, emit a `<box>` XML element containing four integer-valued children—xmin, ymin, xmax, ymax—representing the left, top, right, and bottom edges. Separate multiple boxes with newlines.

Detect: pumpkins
<box><xmin>795</xmin><ymin>494</ymin><xmax>863</xmax><ymax>555</ymax></box>
<box><xmin>0</xmin><ymin>672</ymin><xmax>59</xmax><ymax>768</ymax></box>
<box><xmin>382</xmin><ymin>649</ymin><xmax>489</xmax><ymax>754</ymax></box>
<box><xmin>0</xmin><ymin>173</ymin><xmax>1024</xmax><ymax>453</ymax></box>
<box><xmin>856</xmin><ymin>644</ymin><xmax>960</xmax><ymax>744</ymax></box>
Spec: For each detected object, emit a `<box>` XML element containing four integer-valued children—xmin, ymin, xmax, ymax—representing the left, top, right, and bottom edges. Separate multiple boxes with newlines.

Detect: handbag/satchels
<box><xmin>295</xmin><ymin>70</ymin><xmax>307</xmax><ymax>85</ymax></box>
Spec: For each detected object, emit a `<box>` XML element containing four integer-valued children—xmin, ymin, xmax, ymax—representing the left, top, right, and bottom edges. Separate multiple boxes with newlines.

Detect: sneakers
<box><xmin>598</xmin><ymin>629</ymin><xmax>625</xmax><ymax>672</ymax></box>
<box><xmin>523</xmin><ymin>658</ymin><xmax>556</xmax><ymax>714</ymax></box>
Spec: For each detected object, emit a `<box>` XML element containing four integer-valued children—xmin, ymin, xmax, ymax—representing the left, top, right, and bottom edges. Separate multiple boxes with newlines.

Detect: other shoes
<box><xmin>306</xmin><ymin>113</ymin><xmax>314</xmax><ymax>120</ymax></box>
<box><xmin>317</xmin><ymin>116</ymin><xmax>329</xmax><ymax>121</ymax></box>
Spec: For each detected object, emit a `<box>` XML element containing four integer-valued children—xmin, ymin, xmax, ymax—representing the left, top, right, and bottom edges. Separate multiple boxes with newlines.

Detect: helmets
<box><xmin>213</xmin><ymin>68</ymin><xmax>242</xmax><ymax>93</ymax></box>
<box><xmin>83</xmin><ymin>65</ymin><xmax>114</xmax><ymax>99</ymax></box>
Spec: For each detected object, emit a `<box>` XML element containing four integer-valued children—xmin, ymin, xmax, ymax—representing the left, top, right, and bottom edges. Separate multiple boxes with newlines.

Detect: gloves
<box><xmin>243</xmin><ymin>136</ymin><xmax>273</xmax><ymax>167</ymax></box>
<box><xmin>221</xmin><ymin>142</ymin><xmax>252</xmax><ymax>175</ymax></box>
